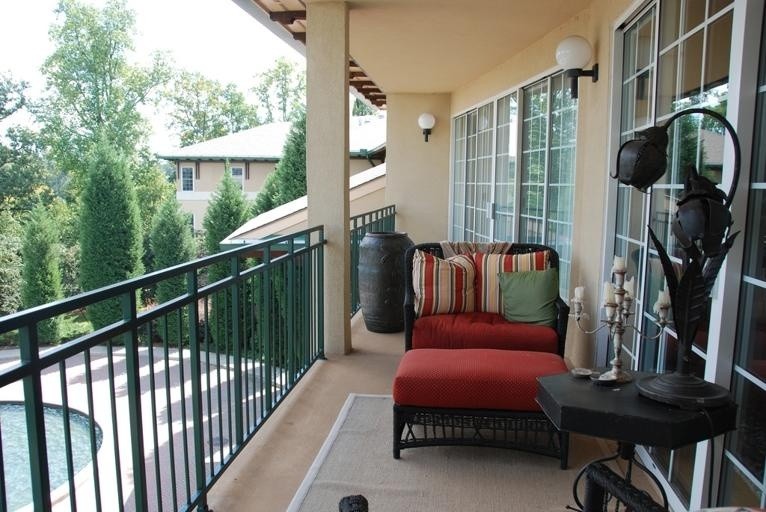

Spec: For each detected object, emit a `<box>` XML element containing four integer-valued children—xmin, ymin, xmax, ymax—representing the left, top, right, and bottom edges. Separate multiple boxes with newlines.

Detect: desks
<box><xmin>536</xmin><ymin>366</ymin><xmax>739</xmax><ymax>512</ymax></box>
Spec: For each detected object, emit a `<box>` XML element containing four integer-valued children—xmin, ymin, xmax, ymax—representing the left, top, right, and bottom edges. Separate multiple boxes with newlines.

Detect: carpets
<box><xmin>284</xmin><ymin>392</ymin><xmax>626</xmax><ymax>511</ymax></box>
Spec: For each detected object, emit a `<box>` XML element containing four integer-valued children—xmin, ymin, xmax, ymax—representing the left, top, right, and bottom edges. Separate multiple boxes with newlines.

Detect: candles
<box><xmin>657</xmin><ymin>290</ymin><xmax>670</xmax><ymax>303</ymax></box>
<box><xmin>603</xmin><ymin>254</ymin><xmax>635</xmax><ymax>303</ymax></box>
<box><xmin>576</xmin><ymin>285</ymin><xmax>585</xmax><ymax>298</ymax></box>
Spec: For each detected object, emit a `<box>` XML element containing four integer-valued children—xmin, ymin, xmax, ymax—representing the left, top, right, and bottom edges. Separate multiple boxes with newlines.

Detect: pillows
<box><xmin>412</xmin><ymin>249</ymin><xmax>560</xmax><ymax>326</ymax></box>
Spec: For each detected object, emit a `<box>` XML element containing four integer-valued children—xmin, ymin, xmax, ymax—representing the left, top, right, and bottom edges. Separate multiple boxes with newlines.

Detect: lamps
<box><xmin>556</xmin><ymin>34</ymin><xmax>599</xmax><ymax>99</ymax></box>
<box><xmin>418</xmin><ymin>113</ymin><xmax>436</xmax><ymax>142</ymax></box>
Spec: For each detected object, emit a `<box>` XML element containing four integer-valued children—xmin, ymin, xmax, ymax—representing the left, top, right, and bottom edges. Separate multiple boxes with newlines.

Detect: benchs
<box><xmin>391</xmin><ymin>348</ymin><xmax>570</xmax><ymax>469</ymax></box>
<box><xmin>404</xmin><ymin>240</ymin><xmax>571</xmax><ymax>359</ymax></box>
